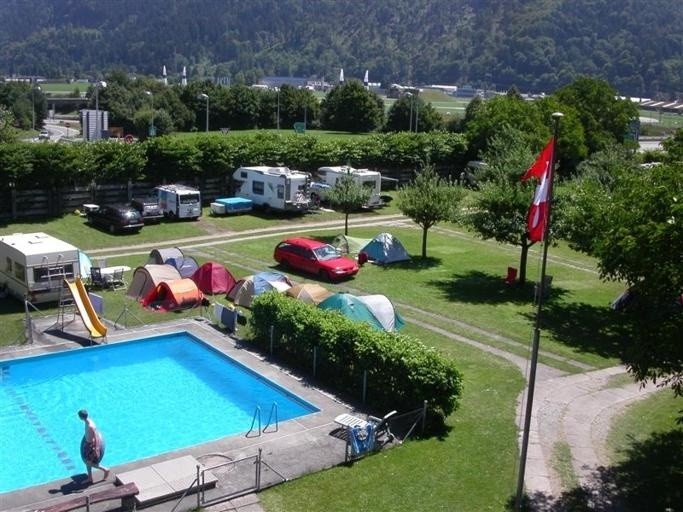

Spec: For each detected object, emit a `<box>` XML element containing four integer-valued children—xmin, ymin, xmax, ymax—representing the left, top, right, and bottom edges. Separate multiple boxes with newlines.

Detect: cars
<box><xmin>274</xmin><ymin>237</ymin><xmax>360</xmax><ymax>281</ymax></box>
<box><xmin>87</xmin><ymin>197</ymin><xmax>164</xmax><ymax>234</ymax></box>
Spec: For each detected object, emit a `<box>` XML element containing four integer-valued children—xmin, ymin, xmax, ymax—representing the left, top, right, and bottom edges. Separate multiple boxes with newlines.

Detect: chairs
<box><xmin>334</xmin><ymin>410</ymin><xmax>398</xmax><ymax>446</ymax></box>
<box><xmin>90</xmin><ymin>258</ymin><xmax>127</xmax><ymax>294</ymax></box>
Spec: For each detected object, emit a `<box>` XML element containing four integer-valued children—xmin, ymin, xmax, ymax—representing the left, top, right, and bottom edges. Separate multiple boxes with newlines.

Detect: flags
<box><xmin>518</xmin><ymin>140</ymin><xmax>555</xmax><ymax>251</ymax></box>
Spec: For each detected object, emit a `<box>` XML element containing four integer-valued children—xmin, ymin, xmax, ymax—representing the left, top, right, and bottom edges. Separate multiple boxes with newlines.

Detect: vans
<box><xmin>453</xmin><ymin>88</ymin><xmax>476</xmax><ymax>96</ymax></box>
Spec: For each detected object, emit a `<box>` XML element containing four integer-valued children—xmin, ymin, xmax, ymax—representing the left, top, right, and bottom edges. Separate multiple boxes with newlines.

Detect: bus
<box><xmin>152</xmin><ymin>184</ymin><xmax>202</xmax><ymax>221</ymax></box>
<box><xmin>316</xmin><ymin>165</ymin><xmax>381</xmax><ymax>211</ymax></box>
<box><xmin>232</xmin><ymin>165</ymin><xmax>312</xmax><ymax>215</ymax></box>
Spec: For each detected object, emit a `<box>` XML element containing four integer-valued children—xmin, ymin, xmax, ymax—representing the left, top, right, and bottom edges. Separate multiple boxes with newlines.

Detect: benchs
<box><xmin>23</xmin><ymin>481</ymin><xmax>140</xmax><ymax>512</ymax></box>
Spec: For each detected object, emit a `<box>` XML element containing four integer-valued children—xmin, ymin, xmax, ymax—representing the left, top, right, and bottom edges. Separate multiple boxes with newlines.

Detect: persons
<box><xmin>77</xmin><ymin>409</ymin><xmax>111</xmax><ymax>480</ymax></box>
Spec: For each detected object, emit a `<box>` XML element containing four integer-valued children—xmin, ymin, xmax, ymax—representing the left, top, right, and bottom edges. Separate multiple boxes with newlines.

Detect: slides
<box><xmin>63</xmin><ymin>275</ymin><xmax>108</xmax><ymax>339</ymax></box>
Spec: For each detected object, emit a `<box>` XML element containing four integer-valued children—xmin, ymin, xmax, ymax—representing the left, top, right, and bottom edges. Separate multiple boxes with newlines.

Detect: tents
<box><xmin>336</xmin><ymin>233</ymin><xmax>371</xmax><ymax>252</ymax></box>
<box><xmin>360</xmin><ymin>232</ymin><xmax>413</xmax><ymax>266</ymax></box>
<box><xmin>128</xmin><ymin>246</ymin><xmax>401</xmax><ymax>338</ymax></box>
<box><xmin>612</xmin><ymin>282</ymin><xmax>644</xmax><ymax>312</ymax></box>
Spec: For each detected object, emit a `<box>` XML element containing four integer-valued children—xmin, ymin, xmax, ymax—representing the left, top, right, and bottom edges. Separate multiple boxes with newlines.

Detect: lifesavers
<box><xmin>81</xmin><ymin>430</ymin><xmax>105</xmax><ymax>465</ymax></box>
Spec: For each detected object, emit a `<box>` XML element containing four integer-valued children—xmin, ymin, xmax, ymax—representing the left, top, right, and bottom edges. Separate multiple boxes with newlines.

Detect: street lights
<box><xmin>33</xmin><ymin>87</ymin><xmax>41</xmax><ymax>129</ymax></box>
<box><xmin>275</xmin><ymin>88</ymin><xmax>280</xmax><ymax>129</ymax></box>
<box><xmin>405</xmin><ymin>92</ymin><xmax>413</xmax><ymax>132</ymax></box>
<box><xmin>145</xmin><ymin>91</ymin><xmax>153</xmax><ymax>138</ymax></box>
<box><xmin>96</xmin><ymin>81</ymin><xmax>107</xmax><ymax>140</ymax></box>
<box><xmin>197</xmin><ymin>93</ymin><xmax>209</xmax><ymax>134</ymax></box>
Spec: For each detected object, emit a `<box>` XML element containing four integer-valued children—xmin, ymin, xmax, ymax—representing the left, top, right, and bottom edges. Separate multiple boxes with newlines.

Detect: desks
<box><xmin>95</xmin><ymin>265</ymin><xmax>131</xmax><ymax>287</ymax></box>
